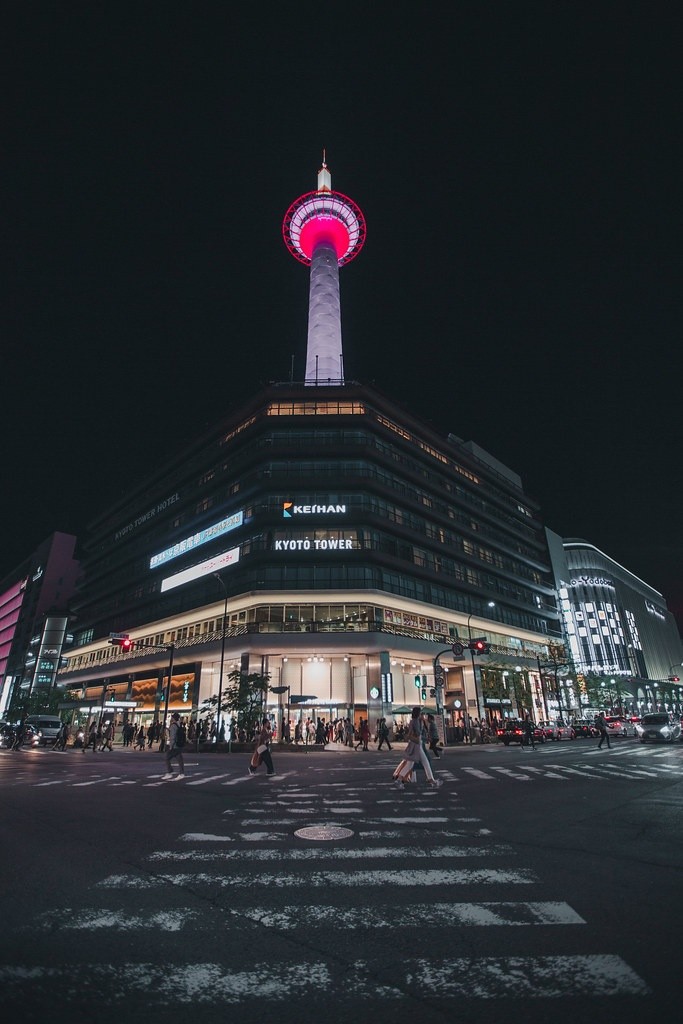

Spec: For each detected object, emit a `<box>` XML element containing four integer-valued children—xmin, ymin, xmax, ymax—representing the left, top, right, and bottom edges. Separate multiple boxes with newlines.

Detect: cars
<box><xmin>626</xmin><ymin>712</ymin><xmax>683</xmax><ymax>744</ymax></box>
<box><xmin>536</xmin><ymin>719</ymin><xmax>576</xmax><ymax>742</ymax></box>
<box><xmin>0</xmin><ymin>722</ymin><xmax>46</xmax><ymax>749</ymax></box>
<box><xmin>604</xmin><ymin>715</ymin><xmax>638</xmax><ymax>738</ymax></box>
<box><xmin>570</xmin><ymin>719</ymin><xmax>600</xmax><ymax>738</ymax></box>
<box><xmin>496</xmin><ymin>719</ymin><xmax>547</xmax><ymax>746</ymax></box>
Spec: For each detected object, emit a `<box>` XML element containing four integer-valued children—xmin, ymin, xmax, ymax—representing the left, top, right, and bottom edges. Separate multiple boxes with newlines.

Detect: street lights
<box><xmin>554</xmin><ymin>663</ymin><xmax>569</xmax><ymax>727</ymax></box>
<box><xmin>213</xmin><ymin>572</ymin><xmax>228</xmax><ymax>743</ymax></box>
<box><xmin>601</xmin><ymin>678</ymin><xmax>616</xmax><ymax>716</ymax></box>
<box><xmin>467</xmin><ymin>601</ymin><xmax>495</xmax><ymax>744</ymax></box>
<box><xmin>645</xmin><ymin>682</ymin><xmax>659</xmax><ymax>712</ymax></box>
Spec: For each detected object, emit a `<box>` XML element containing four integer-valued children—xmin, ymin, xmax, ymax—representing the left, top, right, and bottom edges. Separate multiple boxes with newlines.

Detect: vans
<box><xmin>23</xmin><ymin>714</ymin><xmax>63</xmax><ymax>743</ymax></box>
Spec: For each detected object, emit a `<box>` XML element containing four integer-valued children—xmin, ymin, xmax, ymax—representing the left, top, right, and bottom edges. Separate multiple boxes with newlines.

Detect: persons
<box><xmin>392</xmin><ymin>707</ymin><xmax>443</xmax><ymax>789</ymax></box>
<box><xmin>252</xmin><ymin>717</ymin><xmax>354</xmax><ymax>746</ymax></box>
<box><xmin>354</xmin><ymin>717</ymin><xmax>371</xmax><ymax>751</ymax></box>
<box><xmin>82</xmin><ymin>722</ymin><xmax>114</xmax><ymax>753</ymax></box>
<box><xmin>73</xmin><ymin>726</ymin><xmax>85</xmax><ymax>748</ymax></box>
<box><xmin>247</xmin><ymin>719</ymin><xmax>277</xmax><ymax>777</ymax></box>
<box><xmin>538</xmin><ymin>715</ymin><xmax>580</xmax><ymax>726</ymax></box>
<box><xmin>52</xmin><ymin>724</ymin><xmax>69</xmax><ymax>751</ymax></box>
<box><xmin>227</xmin><ymin>718</ymin><xmax>246</xmax><ymax>742</ymax></box>
<box><xmin>182</xmin><ymin>719</ymin><xmax>226</xmax><ymax>743</ymax></box>
<box><xmin>597</xmin><ymin>713</ymin><xmax>612</xmax><ymax>749</ymax></box>
<box><xmin>374</xmin><ymin>718</ymin><xmax>393</xmax><ymax>751</ymax></box>
<box><xmin>521</xmin><ymin>715</ymin><xmax>537</xmax><ymax>751</ymax></box>
<box><xmin>121</xmin><ymin>719</ymin><xmax>145</xmax><ymax>751</ymax></box>
<box><xmin>12</xmin><ymin>717</ymin><xmax>28</xmax><ymax>752</ymax></box>
<box><xmin>457</xmin><ymin>716</ymin><xmax>519</xmax><ymax>744</ymax></box>
<box><xmin>162</xmin><ymin>713</ymin><xmax>185</xmax><ymax>780</ymax></box>
<box><xmin>147</xmin><ymin>720</ymin><xmax>166</xmax><ymax>751</ymax></box>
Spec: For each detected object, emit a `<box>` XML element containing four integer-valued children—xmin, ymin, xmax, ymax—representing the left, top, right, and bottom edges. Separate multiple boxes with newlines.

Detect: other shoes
<box><xmin>432</xmin><ymin>757</ymin><xmax>441</xmax><ymax>760</ymax></box>
<box><xmin>247</xmin><ymin>765</ymin><xmax>256</xmax><ymax>775</ymax></box>
<box><xmin>431</xmin><ymin>780</ymin><xmax>445</xmax><ymax>789</ymax></box>
<box><xmin>442</xmin><ymin>749</ymin><xmax>444</xmax><ymax>756</ymax></box>
<box><xmin>161</xmin><ymin>773</ymin><xmax>173</xmax><ymax>780</ymax></box>
<box><xmin>394</xmin><ymin>779</ymin><xmax>405</xmax><ymax>789</ymax></box>
<box><xmin>174</xmin><ymin>774</ymin><xmax>185</xmax><ymax>781</ymax></box>
<box><xmin>598</xmin><ymin>745</ymin><xmax>603</xmax><ymax>749</ymax></box>
<box><xmin>608</xmin><ymin>747</ymin><xmax>614</xmax><ymax>749</ymax></box>
<box><xmin>266</xmin><ymin>772</ymin><xmax>277</xmax><ymax>777</ymax></box>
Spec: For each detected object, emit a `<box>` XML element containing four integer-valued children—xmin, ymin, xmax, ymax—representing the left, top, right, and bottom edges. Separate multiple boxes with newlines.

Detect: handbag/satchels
<box><xmin>107</xmin><ymin>740</ymin><xmax>112</xmax><ymax>747</ymax></box>
<box><xmin>408</xmin><ymin>728</ymin><xmax>421</xmax><ymax>745</ymax></box>
<box><xmin>256</xmin><ymin>744</ymin><xmax>268</xmax><ymax>755</ymax></box>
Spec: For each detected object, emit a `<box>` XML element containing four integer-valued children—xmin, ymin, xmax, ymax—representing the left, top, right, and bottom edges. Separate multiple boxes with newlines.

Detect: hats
<box><xmin>172</xmin><ymin>713</ymin><xmax>180</xmax><ymax>721</ymax></box>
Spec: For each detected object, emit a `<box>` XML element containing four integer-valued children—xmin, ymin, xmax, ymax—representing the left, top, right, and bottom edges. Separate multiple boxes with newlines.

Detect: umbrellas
<box><xmin>421</xmin><ymin>707</ymin><xmax>441</xmax><ymax>718</ymax></box>
<box><xmin>392</xmin><ymin>706</ymin><xmax>412</xmax><ymax>724</ymax></box>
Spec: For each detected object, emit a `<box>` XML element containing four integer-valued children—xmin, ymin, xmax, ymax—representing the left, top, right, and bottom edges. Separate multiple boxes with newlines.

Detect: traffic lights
<box><xmin>122</xmin><ymin>639</ymin><xmax>130</xmax><ymax>652</ymax></box>
<box><xmin>161</xmin><ymin>689</ymin><xmax>164</xmax><ymax>701</ymax></box>
<box><xmin>110</xmin><ymin>692</ymin><xmax>115</xmax><ymax>702</ymax></box>
<box><xmin>669</xmin><ymin>677</ymin><xmax>679</xmax><ymax>681</ymax></box>
<box><xmin>475</xmin><ymin>641</ymin><xmax>485</xmax><ymax>650</ymax></box>
<box><xmin>415</xmin><ymin>676</ymin><xmax>420</xmax><ymax>687</ymax></box>
<box><xmin>422</xmin><ymin>689</ymin><xmax>426</xmax><ymax>700</ymax></box>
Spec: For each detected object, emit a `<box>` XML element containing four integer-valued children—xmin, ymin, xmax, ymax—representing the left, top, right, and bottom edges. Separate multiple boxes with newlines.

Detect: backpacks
<box><xmin>175</xmin><ymin>722</ymin><xmax>187</xmax><ymax>747</ymax></box>
<box><xmin>595</xmin><ymin>720</ymin><xmax>602</xmax><ymax>730</ymax></box>
